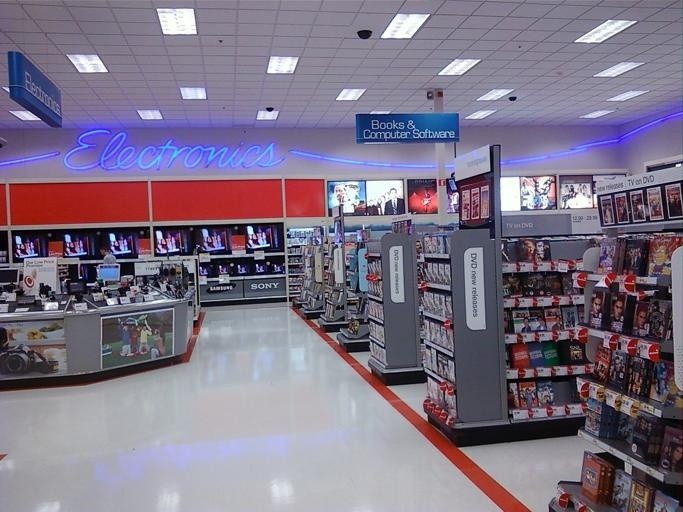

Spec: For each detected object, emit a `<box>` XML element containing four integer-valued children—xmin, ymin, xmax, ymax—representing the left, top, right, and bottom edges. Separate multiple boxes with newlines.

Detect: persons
<box><xmin>562</xmin><ymin>184</ymin><xmax>589</xmax><ymax>208</ymax></box>
<box><xmin>333</xmin><ymin>182</ymin><xmax>345</xmax><ymax>203</ymax></box>
<box><xmin>100</xmin><ymin>246</ymin><xmax>118</xmax><ymax>285</ymax></box>
<box><xmin>502</xmin><ymin>235</ymin><xmax>585</xmax><ymax>333</ymax></box>
<box><xmin>582</xmin><ymin>232</ymin><xmax>681</xmax><ymax>463</ymax></box>
<box><xmin>116</xmin><ymin>315</ymin><xmax>165</xmax><ymax>356</ymax></box>
<box><xmin>367</xmin><ymin>186</ymin><xmax>404</xmax><ymax>213</ymax></box>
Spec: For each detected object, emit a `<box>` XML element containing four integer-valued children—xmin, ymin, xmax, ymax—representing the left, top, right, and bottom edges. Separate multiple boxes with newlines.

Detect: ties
<box><xmin>393</xmin><ymin>199</ymin><xmax>397</xmax><ymax>215</ymax></box>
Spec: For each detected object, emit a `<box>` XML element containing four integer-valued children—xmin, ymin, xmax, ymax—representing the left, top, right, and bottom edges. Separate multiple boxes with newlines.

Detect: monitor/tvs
<box><xmin>11</xmin><ymin>223</ymin><xmax>284</xmax><ymax>286</ymax></box>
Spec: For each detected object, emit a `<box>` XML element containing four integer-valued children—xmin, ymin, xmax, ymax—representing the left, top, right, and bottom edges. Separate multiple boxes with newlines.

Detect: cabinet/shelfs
<box><xmin>548</xmin><ymin>164</ymin><xmax>682</xmax><ymax>511</ymax></box>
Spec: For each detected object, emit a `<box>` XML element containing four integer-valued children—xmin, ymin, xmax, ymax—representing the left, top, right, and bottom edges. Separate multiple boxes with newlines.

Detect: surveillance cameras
<box><xmin>357</xmin><ymin>29</ymin><xmax>373</xmax><ymax>40</ymax></box>
<box><xmin>509</xmin><ymin>97</ymin><xmax>516</xmax><ymax>102</ymax></box>
<box><xmin>265</xmin><ymin>107</ymin><xmax>274</xmax><ymax>112</ymax></box>
